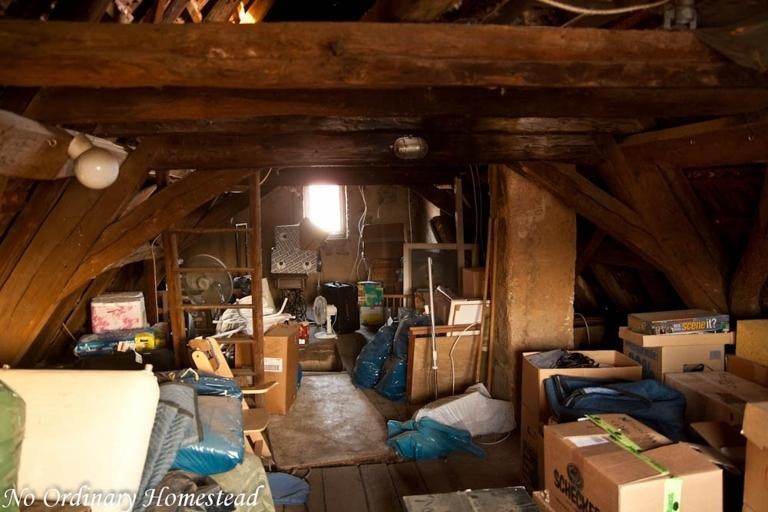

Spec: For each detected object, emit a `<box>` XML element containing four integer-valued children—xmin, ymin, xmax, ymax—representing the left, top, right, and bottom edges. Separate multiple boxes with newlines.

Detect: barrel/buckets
<box><xmin>357</xmin><ymin>279</ymin><xmax>386</xmax><ymax>327</ymax></box>
<box><xmin>323</xmin><ymin>280</ymin><xmax>357</xmax><ymax>332</ymax></box>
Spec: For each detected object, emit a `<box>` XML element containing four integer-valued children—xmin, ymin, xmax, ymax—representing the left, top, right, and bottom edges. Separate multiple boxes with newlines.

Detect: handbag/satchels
<box><xmin>545</xmin><ymin>373</ymin><xmax>689</xmax><ymax>436</ymax></box>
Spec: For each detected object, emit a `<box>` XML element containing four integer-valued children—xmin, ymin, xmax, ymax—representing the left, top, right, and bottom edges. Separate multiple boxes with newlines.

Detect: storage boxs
<box><xmin>258</xmin><ymin>323</ymin><xmax>301</xmax><ymax>416</ymax></box>
<box><xmin>521</xmin><ymin>306</ymin><xmax>768</xmax><ymax>512</ymax></box>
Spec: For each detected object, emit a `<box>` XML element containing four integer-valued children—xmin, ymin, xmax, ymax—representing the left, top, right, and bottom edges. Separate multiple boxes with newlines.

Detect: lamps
<box><xmin>66</xmin><ymin>131</ymin><xmax>120</xmax><ymax>192</ymax></box>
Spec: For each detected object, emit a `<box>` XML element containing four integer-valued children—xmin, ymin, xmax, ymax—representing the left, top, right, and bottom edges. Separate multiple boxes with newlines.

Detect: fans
<box><xmin>312</xmin><ymin>296</ymin><xmax>339</xmax><ymax>340</ymax></box>
<box><xmin>183</xmin><ymin>255</ymin><xmax>234</xmax><ymax>327</ymax></box>
